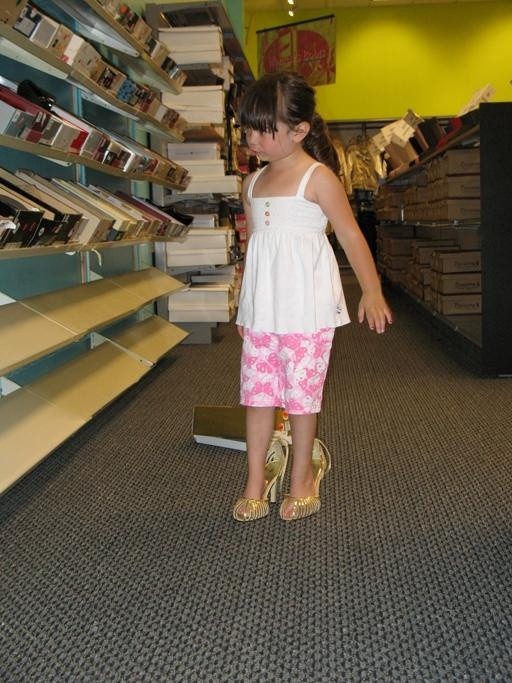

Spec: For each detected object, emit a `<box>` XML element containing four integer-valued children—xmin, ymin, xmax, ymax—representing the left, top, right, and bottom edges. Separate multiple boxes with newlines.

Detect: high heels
<box><xmin>279</xmin><ymin>438</ymin><xmax>332</xmax><ymax>521</ymax></box>
<box><xmin>233</xmin><ymin>436</ymin><xmax>289</xmax><ymax>522</ymax></box>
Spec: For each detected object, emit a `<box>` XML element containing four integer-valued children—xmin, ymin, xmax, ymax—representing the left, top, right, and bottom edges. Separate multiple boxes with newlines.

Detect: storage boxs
<box><xmin>374</xmin><ymin>149</ymin><xmax>484</xmax><ymax>313</ymax></box>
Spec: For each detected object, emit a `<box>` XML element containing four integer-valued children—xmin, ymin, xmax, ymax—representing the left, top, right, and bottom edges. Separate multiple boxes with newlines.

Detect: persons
<box><xmin>235</xmin><ymin>73</ymin><xmax>393</xmax><ymax>521</ymax></box>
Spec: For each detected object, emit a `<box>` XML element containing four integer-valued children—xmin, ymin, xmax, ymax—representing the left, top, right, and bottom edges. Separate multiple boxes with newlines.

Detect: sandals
<box><xmin>16</xmin><ymin>79</ymin><xmax>62</xmax><ymax>122</ymax></box>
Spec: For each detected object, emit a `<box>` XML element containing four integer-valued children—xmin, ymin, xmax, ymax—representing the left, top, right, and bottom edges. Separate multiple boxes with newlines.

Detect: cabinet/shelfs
<box><xmin>144</xmin><ymin>2</ymin><xmax>245</xmax><ymax>347</ymax></box>
<box><xmin>3</xmin><ymin>0</ymin><xmax>190</xmax><ymax>494</ymax></box>
<box><xmin>373</xmin><ymin>101</ymin><xmax>509</xmax><ymax>379</ymax></box>
